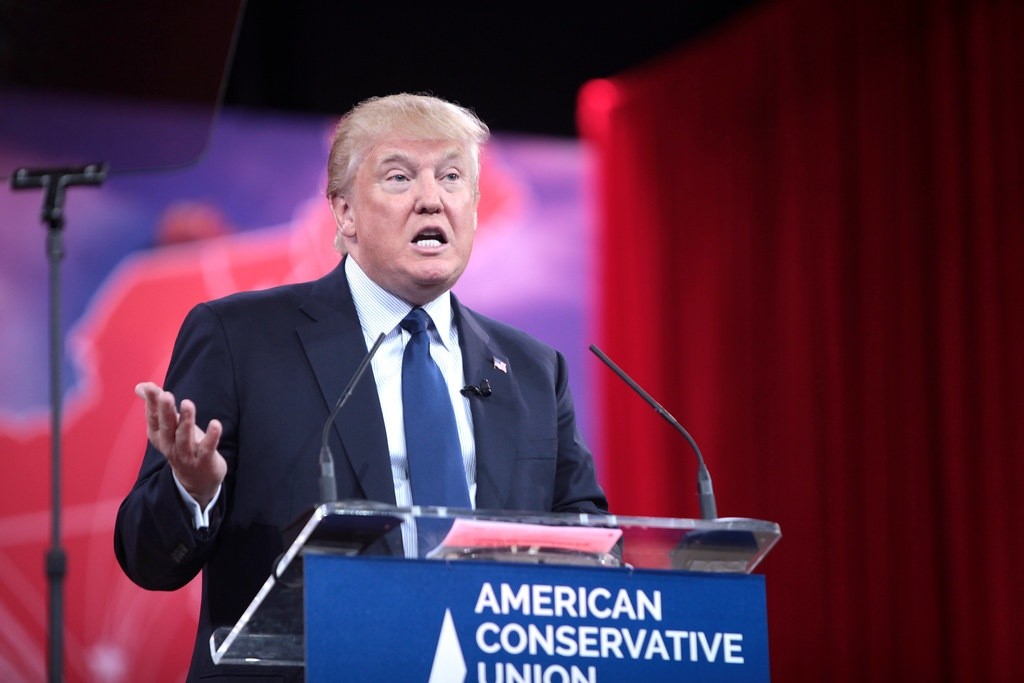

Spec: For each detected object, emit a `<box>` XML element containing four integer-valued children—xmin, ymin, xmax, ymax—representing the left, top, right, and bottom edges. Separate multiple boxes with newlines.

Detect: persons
<box><xmin>114</xmin><ymin>94</ymin><xmax>621</xmax><ymax>683</ymax></box>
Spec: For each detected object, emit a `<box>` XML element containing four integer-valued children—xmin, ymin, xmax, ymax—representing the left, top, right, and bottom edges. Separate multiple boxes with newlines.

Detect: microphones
<box><xmin>589</xmin><ymin>344</ymin><xmax>780</xmax><ymax>572</ymax></box>
<box><xmin>296</xmin><ymin>333</ymin><xmax>406</xmax><ymax>557</ymax></box>
<box><xmin>480</xmin><ymin>378</ymin><xmax>492</xmax><ymax>397</ymax></box>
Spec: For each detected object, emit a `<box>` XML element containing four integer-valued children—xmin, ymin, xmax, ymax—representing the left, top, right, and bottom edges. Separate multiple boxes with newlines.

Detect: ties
<box><xmin>399</xmin><ymin>308</ymin><xmax>473</xmax><ymax>560</ymax></box>
